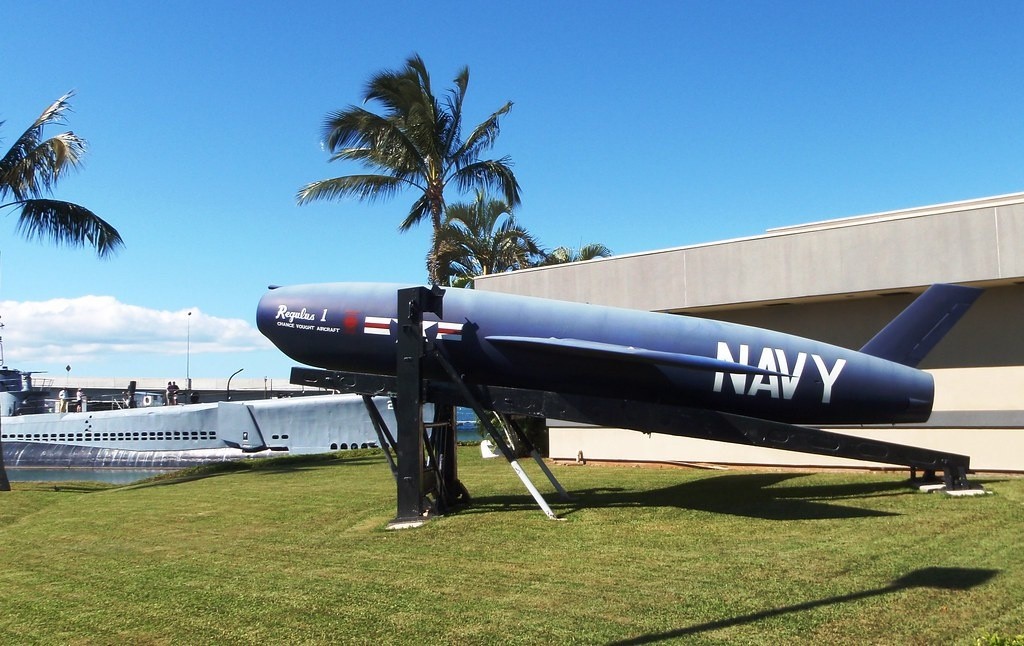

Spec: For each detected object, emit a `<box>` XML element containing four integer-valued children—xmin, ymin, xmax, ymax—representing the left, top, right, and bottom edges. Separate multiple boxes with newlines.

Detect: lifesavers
<box><xmin>143</xmin><ymin>396</ymin><xmax>152</xmax><ymax>406</ymax></box>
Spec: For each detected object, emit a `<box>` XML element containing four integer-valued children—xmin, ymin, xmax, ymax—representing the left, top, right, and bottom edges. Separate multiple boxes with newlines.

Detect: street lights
<box><xmin>186</xmin><ymin>311</ymin><xmax>192</xmax><ymax>378</ymax></box>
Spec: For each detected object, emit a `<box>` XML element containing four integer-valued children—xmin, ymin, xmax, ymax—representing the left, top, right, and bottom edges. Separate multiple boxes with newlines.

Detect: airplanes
<box><xmin>254</xmin><ymin>277</ymin><xmax>988</xmax><ymax>424</ymax></box>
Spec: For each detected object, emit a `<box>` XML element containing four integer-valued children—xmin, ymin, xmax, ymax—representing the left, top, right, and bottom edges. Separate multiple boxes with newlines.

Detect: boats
<box><xmin>0</xmin><ymin>313</ymin><xmax>482</xmax><ymax>472</ymax></box>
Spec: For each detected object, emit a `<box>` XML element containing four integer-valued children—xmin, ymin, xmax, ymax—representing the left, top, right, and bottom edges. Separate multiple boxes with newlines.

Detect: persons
<box><xmin>59</xmin><ymin>386</ymin><xmax>68</xmax><ymax>413</ymax></box>
<box><xmin>166</xmin><ymin>381</ymin><xmax>179</xmax><ymax>405</ymax></box>
<box><xmin>76</xmin><ymin>387</ymin><xmax>83</xmax><ymax>412</ymax></box>
